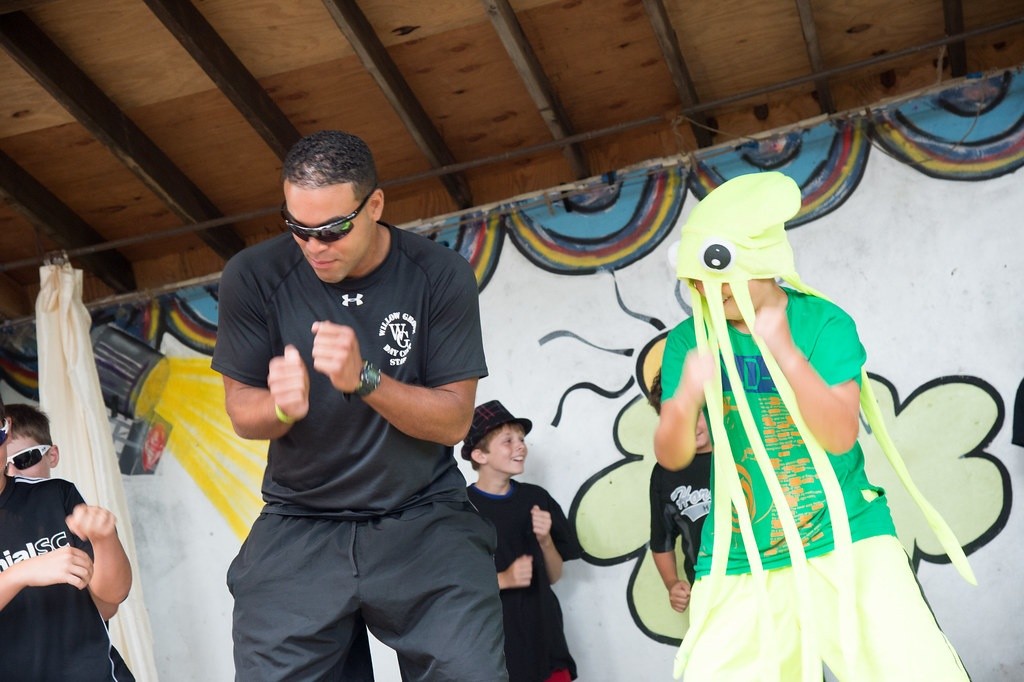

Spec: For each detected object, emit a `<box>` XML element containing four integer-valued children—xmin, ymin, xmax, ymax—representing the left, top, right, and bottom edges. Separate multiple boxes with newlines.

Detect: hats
<box><xmin>460</xmin><ymin>400</ymin><xmax>532</xmax><ymax>461</ymax></box>
<box><xmin>676</xmin><ymin>169</ymin><xmax>801</xmax><ymax>282</ymax></box>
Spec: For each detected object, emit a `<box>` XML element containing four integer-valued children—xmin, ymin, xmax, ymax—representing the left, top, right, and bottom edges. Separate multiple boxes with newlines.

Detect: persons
<box><xmin>650</xmin><ymin>365</ymin><xmax>712</xmax><ymax>613</ymax></box>
<box><xmin>0</xmin><ymin>393</ymin><xmax>135</xmax><ymax>682</ymax></box>
<box><xmin>210</xmin><ymin>131</ymin><xmax>509</xmax><ymax>682</ymax></box>
<box><xmin>463</xmin><ymin>400</ymin><xmax>582</xmax><ymax>682</ymax></box>
<box><xmin>652</xmin><ymin>170</ymin><xmax>970</xmax><ymax>682</ymax></box>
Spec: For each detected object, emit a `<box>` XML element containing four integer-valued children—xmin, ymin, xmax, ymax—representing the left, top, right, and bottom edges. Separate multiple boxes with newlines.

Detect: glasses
<box><xmin>0</xmin><ymin>418</ymin><xmax>9</xmax><ymax>445</ymax></box>
<box><xmin>280</xmin><ymin>183</ymin><xmax>379</xmax><ymax>242</ymax></box>
<box><xmin>5</xmin><ymin>444</ymin><xmax>51</xmax><ymax>474</ymax></box>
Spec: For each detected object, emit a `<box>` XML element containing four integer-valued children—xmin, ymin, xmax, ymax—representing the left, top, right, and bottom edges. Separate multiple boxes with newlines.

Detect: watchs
<box><xmin>342</xmin><ymin>359</ymin><xmax>381</xmax><ymax>402</ymax></box>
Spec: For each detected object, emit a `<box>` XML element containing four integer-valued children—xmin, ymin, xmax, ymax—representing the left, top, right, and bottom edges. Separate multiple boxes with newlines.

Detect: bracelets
<box><xmin>275</xmin><ymin>404</ymin><xmax>296</xmax><ymax>424</ymax></box>
